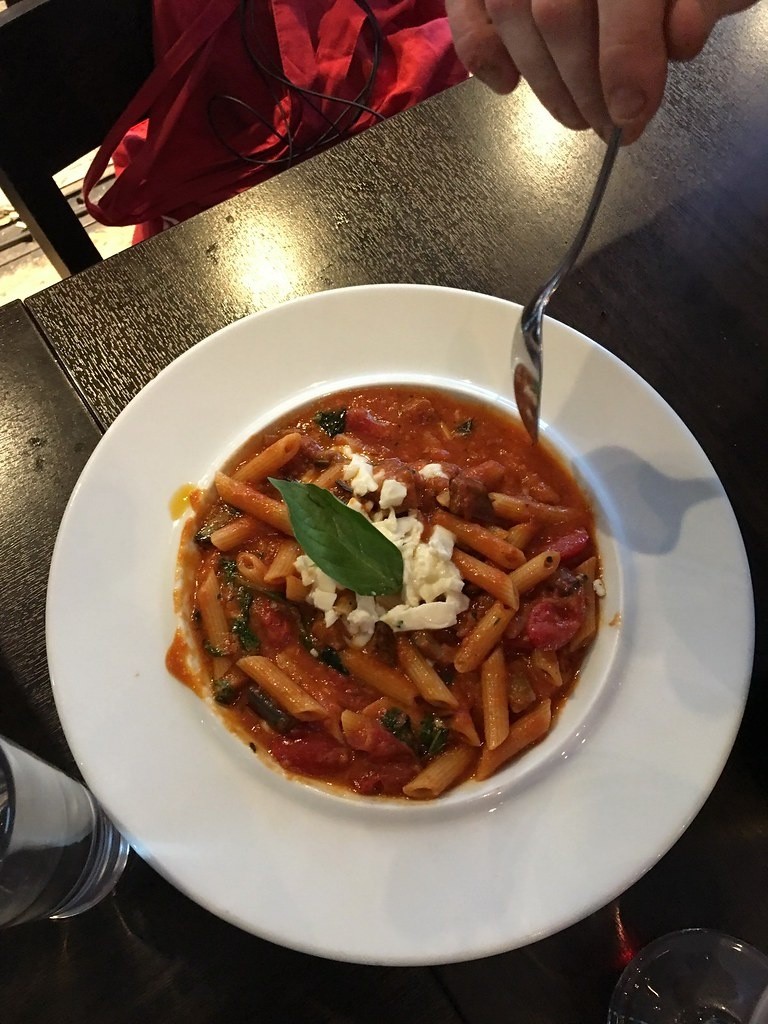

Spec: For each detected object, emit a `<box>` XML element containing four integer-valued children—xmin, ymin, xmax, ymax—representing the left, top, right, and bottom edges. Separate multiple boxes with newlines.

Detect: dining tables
<box><xmin>0</xmin><ymin>0</ymin><xmax>768</xmax><ymax>1024</ymax></box>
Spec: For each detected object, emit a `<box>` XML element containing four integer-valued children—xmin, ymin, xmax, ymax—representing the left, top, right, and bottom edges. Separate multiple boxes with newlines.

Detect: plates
<box><xmin>45</xmin><ymin>284</ymin><xmax>755</xmax><ymax>966</ymax></box>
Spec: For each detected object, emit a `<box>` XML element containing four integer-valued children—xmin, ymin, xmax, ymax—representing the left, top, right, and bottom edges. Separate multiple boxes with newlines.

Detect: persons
<box><xmin>446</xmin><ymin>0</ymin><xmax>762</xmax><ymax>146</ymax></box>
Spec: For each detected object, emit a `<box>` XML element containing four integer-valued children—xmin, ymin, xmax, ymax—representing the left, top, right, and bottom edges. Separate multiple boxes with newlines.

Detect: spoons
<box><xmin>510</xmin><ymin>122</ymin><xmax>621</xmax><ymax>447</ymax></box>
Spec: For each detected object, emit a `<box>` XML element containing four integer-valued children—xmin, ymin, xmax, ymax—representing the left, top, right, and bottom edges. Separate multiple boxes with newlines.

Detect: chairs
<box><xmin>0</xmin><ymin>0</ymin><xmax>154</xmax><ymax>279</ymax></box>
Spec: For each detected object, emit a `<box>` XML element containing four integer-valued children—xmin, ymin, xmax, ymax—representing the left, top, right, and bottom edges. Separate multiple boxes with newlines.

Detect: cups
<box><xmin>0</xmin><ymin>737</ymin><xmax>129</xmax><ymax>928</ymax></box>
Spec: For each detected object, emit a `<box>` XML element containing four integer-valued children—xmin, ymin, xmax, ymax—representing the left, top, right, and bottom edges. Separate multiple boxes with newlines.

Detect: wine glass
<box><xmin>607</xmin><ymin>930</ymin><xmax>768</xmax><ymax>1024</ymax></box>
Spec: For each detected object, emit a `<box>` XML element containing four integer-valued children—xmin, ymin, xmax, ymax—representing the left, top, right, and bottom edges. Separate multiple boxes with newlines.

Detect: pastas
<box><xmin>192</xmin><ymin>432</ymin><xmax>595</xmax><ymax>801</ymax></box>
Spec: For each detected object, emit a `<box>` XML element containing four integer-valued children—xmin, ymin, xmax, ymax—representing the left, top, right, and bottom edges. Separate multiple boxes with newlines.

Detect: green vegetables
<box><xmin>191</xmin><ymin>405</ymin><xmax>457</xmax><ymax>762</ymax></box>
